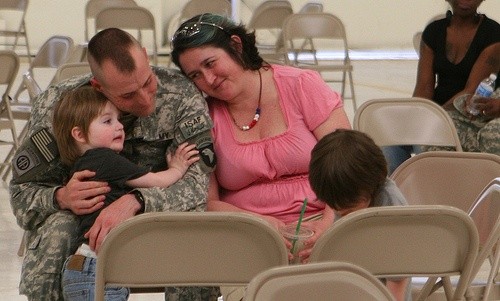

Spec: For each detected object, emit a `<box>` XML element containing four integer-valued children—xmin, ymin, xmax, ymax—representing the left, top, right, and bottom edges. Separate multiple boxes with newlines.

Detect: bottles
<box><xmin>465</xmin><ymin>74</ymin><xmax>497</xmax><ymax>115</ymax></box>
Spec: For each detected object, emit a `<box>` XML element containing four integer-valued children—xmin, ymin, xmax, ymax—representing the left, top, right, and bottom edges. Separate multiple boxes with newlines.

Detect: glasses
<box><xmin>170</xmin><ymin>21</ymin><xmax>229</xmax><ymax>48</ymax></box>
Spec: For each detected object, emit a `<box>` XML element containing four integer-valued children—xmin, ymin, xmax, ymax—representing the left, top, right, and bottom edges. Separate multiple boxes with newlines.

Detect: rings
<box><xmin>483</xmin><ymin>110</ymin><xmax>486</xmax><ymax>115</ymax></box>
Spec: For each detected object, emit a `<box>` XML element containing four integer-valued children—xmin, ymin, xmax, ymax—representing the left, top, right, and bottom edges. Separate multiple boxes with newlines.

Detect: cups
<box><xmin>279</xmin><ymin>224</ymin><xmax>314</xmax><ymax>264</ymax></box>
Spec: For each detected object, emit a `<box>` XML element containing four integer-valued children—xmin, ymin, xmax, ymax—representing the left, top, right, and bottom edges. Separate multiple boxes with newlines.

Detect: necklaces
<box><xmin>225</xmin><ymin>68</ymin><xmax>262</xmax><ymax>131</ymax></box>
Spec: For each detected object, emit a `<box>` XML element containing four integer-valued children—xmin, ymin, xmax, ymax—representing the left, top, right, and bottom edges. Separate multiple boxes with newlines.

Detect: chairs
<box><xmin>0</xmin><ymin>0</ymin><xmax>500</xmax><ymax>301</ymax></box>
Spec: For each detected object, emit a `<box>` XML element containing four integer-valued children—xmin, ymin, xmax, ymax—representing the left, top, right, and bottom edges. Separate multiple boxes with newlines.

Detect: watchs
<box><xmin>134</xmin><ymin>190</ymin><xmax>145</xmax><ymax>212</ymax></box>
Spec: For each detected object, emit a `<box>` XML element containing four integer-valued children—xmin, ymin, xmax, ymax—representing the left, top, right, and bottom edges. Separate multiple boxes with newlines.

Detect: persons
<box><xmin>309</xmin><ymin>128</ymin><xmax>412</xmax><ymax>301</ymax></box>
<box><xmin>8</xmin><ymin>27</ymin><xmax>221</xmax><ymax>301</ymax></box>
<box><xmin>379</xmin><ymin>0</ymin><xmax>500</xmax><ymax>175</ymax></box>
<box><xmin>414</xmin><ymin>92</ymin><xmax>500</xmax><ymax>156</ymax></box>
<box><xmin>54</xmin><ymin>85</ymin><xmax>200</xmax><ymax>301</ymax></box>
<box><xmin>171</xmin><ymin>13</ymin><xmax>352</xmax><ymax>266</ymax></box>
